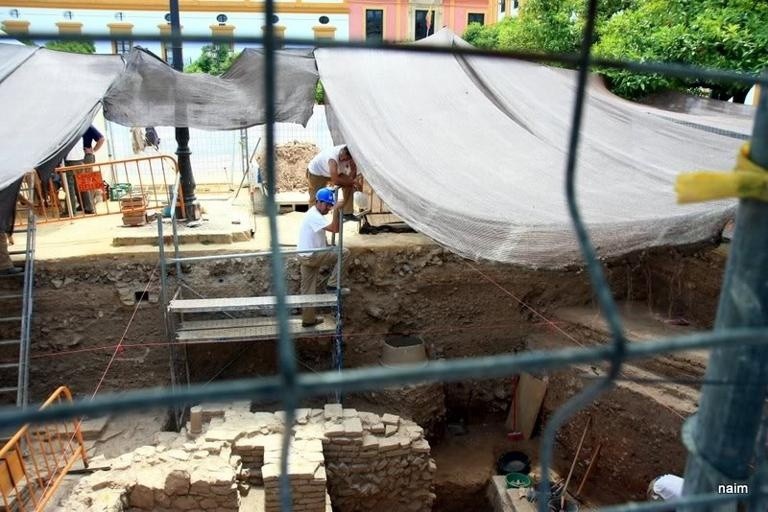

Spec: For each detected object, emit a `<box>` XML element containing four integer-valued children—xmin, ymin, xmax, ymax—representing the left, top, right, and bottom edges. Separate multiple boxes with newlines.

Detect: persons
<box><xmin>76</xmin><ymin>124</ymin><xmax>102</xmax><ymax>211</ymax></box>
<box><xmin>58</xmin><ymin>137</ymin><xmax>95</xmax><ymax>218</ymax></box>
<box><xmin>307</xmin><ymin>143</ymin><xmax>359</xmax><ymax>222</ymax></box>
<box><xmin>0</xmin><ymin>231</ymin><xmax>23</xmax><ymax>275</ymax></box>
<box><xmin>296</xmin><ymin>186</ymin><xmax>354</xmax><ymax>326</ymax></box>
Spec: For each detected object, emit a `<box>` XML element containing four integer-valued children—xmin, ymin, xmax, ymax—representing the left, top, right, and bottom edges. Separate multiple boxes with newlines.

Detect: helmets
<box><xmin>314</xmin><ymin>188</ymin><xmax>335</xmax><ymax>205</ymax></box>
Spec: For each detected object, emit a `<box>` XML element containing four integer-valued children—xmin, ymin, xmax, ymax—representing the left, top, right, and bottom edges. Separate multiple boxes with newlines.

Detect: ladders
<box><xmin>0</xmin><ymin>173</ymin><xmax>37</xmax><ymax>408</ymax></box>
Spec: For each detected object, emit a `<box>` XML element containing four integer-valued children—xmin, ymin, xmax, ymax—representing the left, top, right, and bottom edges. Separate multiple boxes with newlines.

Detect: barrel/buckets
<box><xmin>506</xmin><ymin>472</ymin><xmax>531</xmax><ymax>489</ymax></box>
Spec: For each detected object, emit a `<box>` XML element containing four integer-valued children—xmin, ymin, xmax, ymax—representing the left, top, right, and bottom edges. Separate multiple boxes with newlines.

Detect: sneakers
<box><xmin>342</xmin><ymin>213</ymin><xmax>362</xmax><ymax>221</ymax></box>
<box><xmin>301</xmin><ymin>316</ymin><xmax>325</xmax><ymax>328</ymax></box>
<box><xmin>0</xmin><ymin>266</ymin><xmax>23</xmax><ymax>275</ymax></box>
<box><xmin>326</xmin><ymin>285</ymin><xmax>351</xmax><ymax>294</ymax></box>
<box><xmin>76</xmin><ymin>206</ymin><xmax>96</xmax><ymax>215</ymax></box>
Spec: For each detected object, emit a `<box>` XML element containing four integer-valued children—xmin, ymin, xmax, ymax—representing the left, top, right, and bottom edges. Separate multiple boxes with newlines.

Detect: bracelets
<box><xmin>92</xmin><ymin>148</ymin><xmax>94</xmax><ymax>155</ymax></box>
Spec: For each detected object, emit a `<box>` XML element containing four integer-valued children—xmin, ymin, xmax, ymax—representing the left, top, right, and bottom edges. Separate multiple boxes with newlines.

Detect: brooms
<box><xmin>508</xmin><ymin>375</ymin><xmax>524</xmax><ymax>441</ymax></box>
